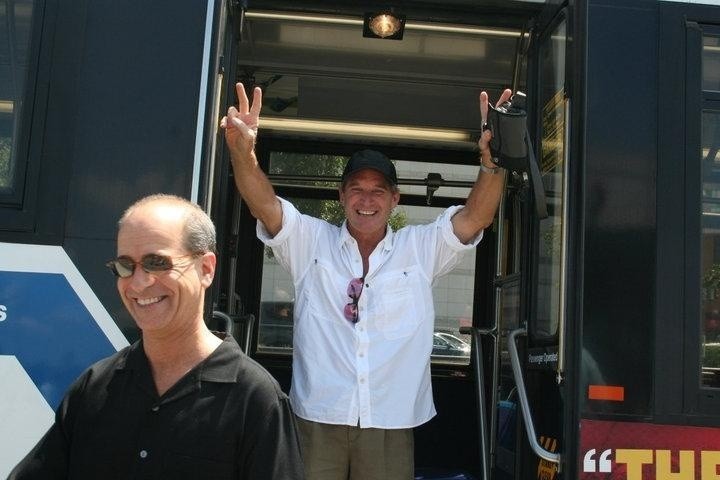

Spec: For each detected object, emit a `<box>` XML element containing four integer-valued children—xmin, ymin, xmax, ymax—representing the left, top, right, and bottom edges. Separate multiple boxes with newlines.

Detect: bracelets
<box><xmin>476</xmin><ymin>159</ymin><xmax>504</xmax><ymax>175</ymax></box>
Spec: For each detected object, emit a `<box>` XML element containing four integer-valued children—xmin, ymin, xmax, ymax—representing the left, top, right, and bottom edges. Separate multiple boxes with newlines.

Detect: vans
<box><xmin>258</xmin><ymin>301</ymin><xmax>293</xmax><ymax>346</ymax></box>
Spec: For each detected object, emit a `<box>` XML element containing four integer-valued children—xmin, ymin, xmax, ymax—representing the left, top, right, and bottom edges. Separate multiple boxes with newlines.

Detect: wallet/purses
<box><xmin>482</xmin><ymin>91</ymin><xmax>529</xmax><ymax>171</ymax></box>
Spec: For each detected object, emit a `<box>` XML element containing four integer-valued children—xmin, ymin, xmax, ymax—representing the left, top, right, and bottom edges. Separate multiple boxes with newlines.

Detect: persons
<box><xmin>218</xmin><ymin>81</ymin><xmax>519</xmax><ymax>479</ymax></box>
<box><xmin>5</xmin><ymin>193</ymin><xmax>309</xmax><ymax>480</ymax></box>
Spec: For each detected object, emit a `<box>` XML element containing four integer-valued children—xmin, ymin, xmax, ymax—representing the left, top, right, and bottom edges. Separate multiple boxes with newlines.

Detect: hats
<box><xmin>342</xmin><ymin>150</ymin><xmax>397</xmax><ymax>185</ymax></box>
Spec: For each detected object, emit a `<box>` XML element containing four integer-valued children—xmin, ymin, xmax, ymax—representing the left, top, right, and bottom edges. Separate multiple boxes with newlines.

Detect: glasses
<box><xmin>106</xmin><ymin>253</ymin><xmax>204</xmax><ymax>278</ymax></box>
<box><xmin>344</xmin><ymin>278</ymin><xmax>364</xmax><ymax>323</ymax></box>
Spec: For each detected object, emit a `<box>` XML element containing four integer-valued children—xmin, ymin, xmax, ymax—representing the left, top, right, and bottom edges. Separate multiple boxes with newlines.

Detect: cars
<box><xmin>432</xmin><ymin>331</ymin><xmax>470</xmax><ymax>356</ymax></box>
